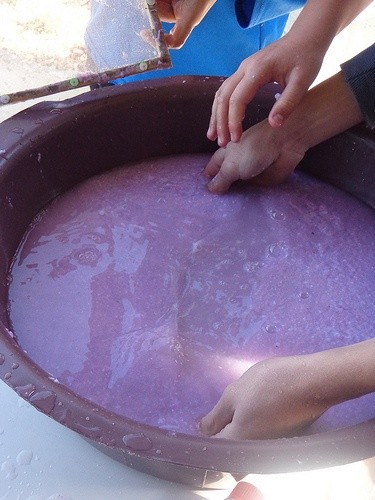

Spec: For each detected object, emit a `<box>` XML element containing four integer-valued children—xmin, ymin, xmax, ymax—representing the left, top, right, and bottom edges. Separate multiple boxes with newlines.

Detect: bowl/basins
<box><xmin>1</xmin><ymin>76</ymin><xmax>374</xmax><ymax>483</ymax></box>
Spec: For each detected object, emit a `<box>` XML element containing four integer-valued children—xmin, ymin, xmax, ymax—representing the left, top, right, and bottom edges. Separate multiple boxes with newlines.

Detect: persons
<box><xmin>206</xmin><ymin>0</ymin><xmax>375</xmax><ymax>146</ymax></box>
<box><xmin>84</xmin><ymin>0</ymin><xmax>306</xmax><ymax>90</ymax></box>
<box><xmin>198</xmin><ymin>44</ymin><xmax>374</xmax><ymax>440</ymax></box>
